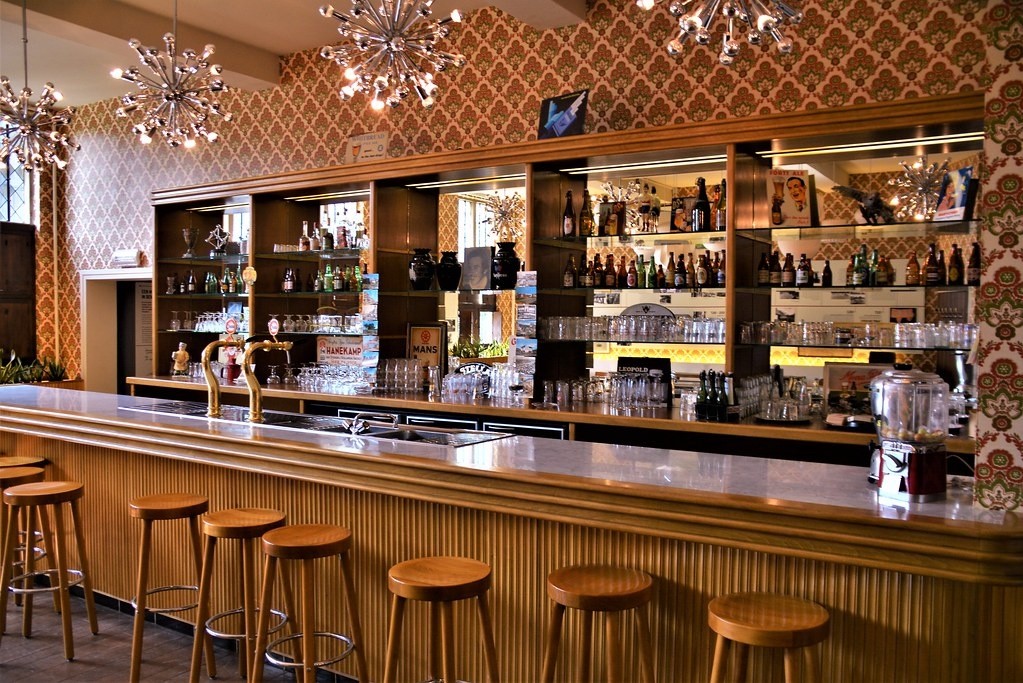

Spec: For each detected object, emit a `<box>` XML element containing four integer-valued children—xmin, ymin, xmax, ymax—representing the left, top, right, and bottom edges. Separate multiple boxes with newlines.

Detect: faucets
<box><xmin>241</xmin><ymin>338</ymin><xmax>293</xmax><ymax>420</ymax></box>
<box><xmin>341</xmin><ymin>412</ymin><xmax>399</xmax><ymax>436</ymax></box>
<box><xmin>200</xmin><ymin>339</ymin><xmax>245</xmax><ymax>416</ymax></box>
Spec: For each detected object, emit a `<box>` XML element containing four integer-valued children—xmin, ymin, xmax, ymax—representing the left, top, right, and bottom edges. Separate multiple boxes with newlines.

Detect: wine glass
<box><xmin>169</xmin><ymin>310</ymin><xmax>249</xmax><ymax>333</ymax></box>
<box><xmin>268</xmin><ymin>314</ymin><xmax>314</xmax><ymax>333</ymax></box>
<box><xmin>266</xmin><ymin>362</ymin><xmax>371</xmax><ymax>396</ymax></box>
<box><xmin>182</xmin><ymin>228</ymin><xmax>200</xmax><ymax>258</ymax></box>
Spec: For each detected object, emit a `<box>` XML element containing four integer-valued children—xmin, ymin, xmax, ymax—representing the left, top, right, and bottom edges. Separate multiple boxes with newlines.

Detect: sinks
<box><xmin>366</xmin><ymin>424</ymin><xmax>514</xmax><ymax>448</ymax></box>
<box><xmin>314</xmin><ymin>423</ymin><xmax>401</xmax><ymax>438</ymax></box>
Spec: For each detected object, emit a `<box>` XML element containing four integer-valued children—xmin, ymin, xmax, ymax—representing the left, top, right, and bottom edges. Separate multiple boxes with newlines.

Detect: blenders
<box><xmin>868</xmin><ymin>362</ymin><xmax>950</xmax><ymax>505</ymax></box>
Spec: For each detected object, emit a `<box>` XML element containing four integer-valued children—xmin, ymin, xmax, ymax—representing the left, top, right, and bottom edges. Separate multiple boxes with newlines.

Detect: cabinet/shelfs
<box><xmin>150</xmin><ymin>92</ymin><xmax>985</xmax><ymax>473</ymax></box>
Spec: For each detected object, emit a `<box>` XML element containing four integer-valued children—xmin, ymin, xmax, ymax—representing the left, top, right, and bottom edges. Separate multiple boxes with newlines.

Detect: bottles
<box><xmin>563</xmin><ymin>249</ymin><xmax>726</xmax><ymax>290</ymax></box>
<box><xmin>562</xmin><ymin>191</ymin><xmax>577</xmax><ymax>238</ymax></box>
<box><xmin>436</xmin><ymin>251</ymin><xmax>462</xmax><ymax>291</ymax></box>
<box><xmin>491</xmin><ymin>242</ymin><xmax>520</xmax><ymax>290</ymax></box>
<box><xmin>579</xmin><ymin>188</ymin><xmax>595</xmax><ymax>236</ymax></box>
<box><xmin>716</xmin><ymin>178</ymin><xmax>726</xmax><ymax>231</ymax></box>
<box><xmin>172</xmin><ymin>268</ymin><xmax>243</xmax><ymax>294</ymax></box>
<box><xmin>281</xmin><ymin>217</ymin><xmax>364</xmax><ymax>294</ymax></box>
<box><xmin>690</xmin><ymin>178</ymin><xmax>710</xmax><ymax>231</ymax></box>
<box><xmin>408</xmin><ymin>248</ymin><xmax>436</xmax><ymax>290</ymax></box>
<box><xmin>709</xmin><ymin>184</ymin><xmax>721</xmax><ymax>231</ymax></box>
<box><xmin>758</xmin><ymin>243</ymin><xmax>981</xmax><ymax>288</ymax></box>
<box><xmin>695</xmin><ymin>369</ymin><xmax>728</xmax><ymax>423</ymax></box>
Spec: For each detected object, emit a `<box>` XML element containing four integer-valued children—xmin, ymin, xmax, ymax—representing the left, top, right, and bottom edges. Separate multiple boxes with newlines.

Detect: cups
<box><xmin>680</xmin><ymin>390</ymin><xmax>698</xmax><ymax>417</ymax></box>
<box><xmin>373</xmin><ymin>358</ymin><xmax>535</xmax><ymax>407</ymax></box>
<box><xmin>543</xmin><ymin>375</ymin><xmax>664</xmax><ymax>409</ymax></box>
<box><xmin>315</xmin><ymin>315</ymin><xmax>362</xmax><ymax>334</ymax></box>
<box><xmin>734</xmin><ymin>318</ymin><xmax>972</xmax><ymax>432</ymax></box>
<box><xmin>187</xmin><ymin>361</ymin><xmax>256</xmax><ymax>384</ymax></box>
<box><xmin>548</xmin><ymin>315</ymin><xmax>725</xmax><ymax>344</ymax></box>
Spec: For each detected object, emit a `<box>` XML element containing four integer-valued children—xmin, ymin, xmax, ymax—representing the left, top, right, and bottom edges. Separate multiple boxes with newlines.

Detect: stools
<box><xmin>130</xmin><ymin>493</ymin><xmax>828</xmax><ymax>683</ymax></box>
<box><xmin>0</xmin><ymin>454</ymin><xmax>99</xmax><ymax>661</ymax></box>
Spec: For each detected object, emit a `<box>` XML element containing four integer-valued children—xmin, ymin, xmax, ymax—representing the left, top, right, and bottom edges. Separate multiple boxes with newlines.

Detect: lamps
<box><xmin>111</xmin><ymin>0</ymin><xmax>233</xmax><ymax>148</ymax></box>
<box><xmin>0</xmin><ymin>0</ymin><xmax>82</xmax><ymax>171</ymax></box>
<box><xmin>636</xmin><ymin>0</ymin><xmax>803</xmax><ymax>64</ymax></box>
<box><xmin>319</xmin><ymin>0</ymin><xmax>466</xmax><ymax>111</ymax></box>
